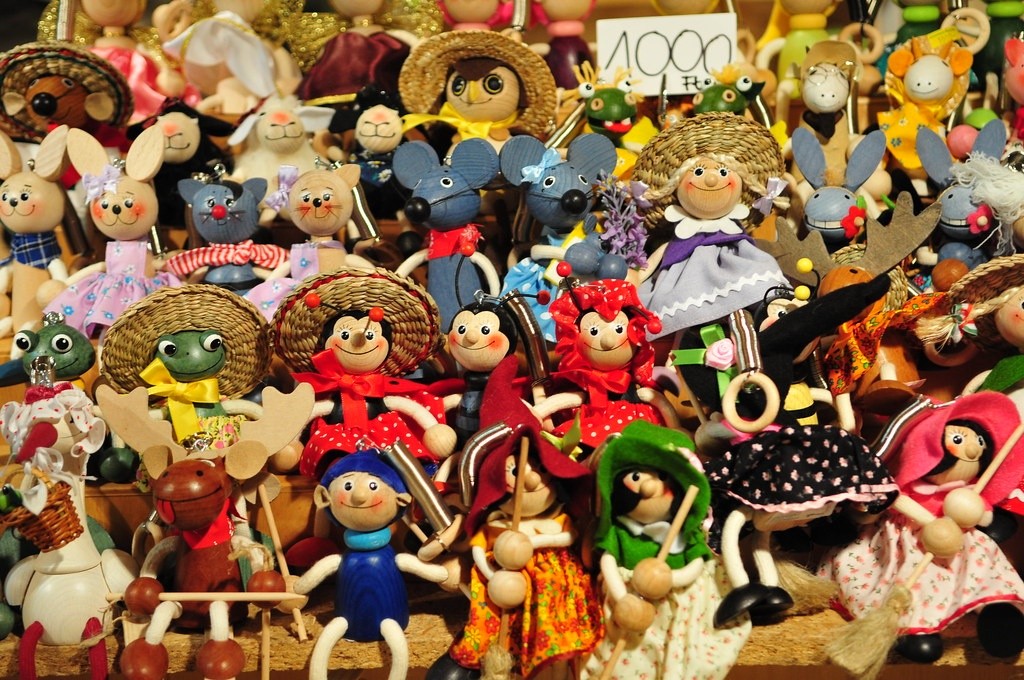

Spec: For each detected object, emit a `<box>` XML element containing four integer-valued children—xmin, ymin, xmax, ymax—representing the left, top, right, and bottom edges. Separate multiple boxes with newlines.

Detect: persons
<box><xmin>956</xmin><ymin>282</ymin><xmax>1024</xmax><ymax>418</ymax></box>
<box><xmin>624</xmin><ymin>153</ymin><xmax>785</xmax><ymax>372</ymax></box>
<box><xmin>294</xmin><ymin>451</ymin><xmax>448</xmax><ymax>679</ymax></box>
<box><xmin>597</xmin><ymin>420</ymin><xmax>714</xmax><ymax>679</ymax></box>
<box><xmin>463</xmin><ymin>425</ymin><xmax>593</xmax><ymax>680</ymax></box>
<box><xmin>828</xmin><ymin>389</ymin><xmax>1023</xmax><ymax>668</ymax></box>
